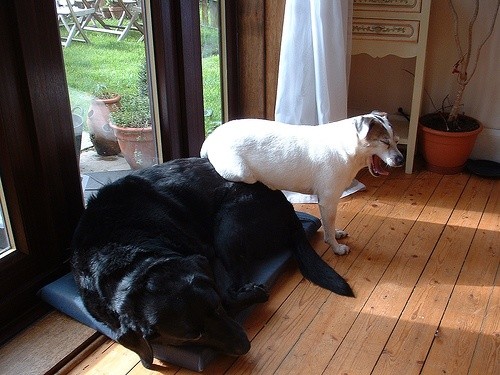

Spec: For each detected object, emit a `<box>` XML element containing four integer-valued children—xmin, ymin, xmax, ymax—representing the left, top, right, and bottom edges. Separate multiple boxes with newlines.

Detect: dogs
<box><xmin>200</xmin><ymin>110</ymin><xmax>405</xmax><ymax>255</ymax></box>
<box><xmin>72</xmin><ymin>157</ymin><xmax>356</xmax><ymax>372</ymax></box>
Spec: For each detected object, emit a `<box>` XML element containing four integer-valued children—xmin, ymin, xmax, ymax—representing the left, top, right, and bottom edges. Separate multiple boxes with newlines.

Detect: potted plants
<box><xmin>102</xmin><ymin>0</ymin><xmax>112</xmax><ymax>19</ymax></box>
<box><xmin>400</xmin><ymin>0</ymin><xmax>500</xmax><ymax>175</ymax></box>
<box><xmin>108</xmin><ymin>55</ymin><xmax>157</xmax><ymax>172</ymax></box>
<box><xmin>86</xmin><ymin>82</ymin><xmax>123</xmax><ymax>156</ymax></box>
<box><xmin>108</xmin><ymin>0</ymin><xmax>124</xmax><ymax>20</ymax></box>
<box><xmin>72</xmin><ymin>106</ymin><xmax>84</xmax><ymax>164</ymax></box>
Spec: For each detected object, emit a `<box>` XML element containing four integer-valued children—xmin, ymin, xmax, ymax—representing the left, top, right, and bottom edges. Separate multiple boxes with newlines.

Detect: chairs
<box><xmin>55</xmin><ymin>0</ymin><xmax>145</xmax><ymax>48</ymax></box>
